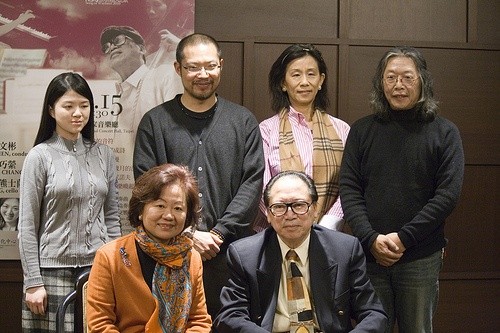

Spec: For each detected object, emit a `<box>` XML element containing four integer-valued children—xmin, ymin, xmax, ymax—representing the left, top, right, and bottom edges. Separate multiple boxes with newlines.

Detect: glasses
<box><xmin>269</xmin><ymin>200</ymin><xmax>314</xmax><ymax>217</ymax></box>
<box><xmin>102</xmin><ymin>35</ymin><xmax>134</xmax><ymax>54</ymax></box>
<box><xmin>183</xmin><ymin>64</ymin><xmax>220</xmax><ymax>73</ymax></box>
<box><xmin>384</xmin><ymin>77</ymin><xmax>419</xmax><ymax>85</ymax></box>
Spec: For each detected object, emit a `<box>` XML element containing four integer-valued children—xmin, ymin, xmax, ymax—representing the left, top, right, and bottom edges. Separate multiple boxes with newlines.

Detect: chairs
<box><xmin>56</xmin><ymin>271</ymin><xmax>90</xmax><ymax>333</ymax></box>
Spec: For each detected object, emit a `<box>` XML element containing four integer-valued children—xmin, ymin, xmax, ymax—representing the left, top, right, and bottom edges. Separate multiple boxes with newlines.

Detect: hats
<box><xmin>99</xmin><ymin>26</ymin><xmax>145</xmax><ymax>45</ymax></box>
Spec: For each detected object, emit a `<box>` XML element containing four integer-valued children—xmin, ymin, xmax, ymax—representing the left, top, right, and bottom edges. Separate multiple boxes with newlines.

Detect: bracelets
<box><xmin>210</xmin><ymin>230</ymin><xmax>225</xmax><ymax>242</ymax></box>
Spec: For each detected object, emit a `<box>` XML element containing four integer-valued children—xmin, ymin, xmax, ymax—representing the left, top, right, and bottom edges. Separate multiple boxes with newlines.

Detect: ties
<box><xmin>285</xmin><ymin>250</ymin><xmax>314</xmax><ymax>333</ymax></box>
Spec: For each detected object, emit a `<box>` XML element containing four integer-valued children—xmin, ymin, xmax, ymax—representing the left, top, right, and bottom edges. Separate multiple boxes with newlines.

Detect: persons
<box><xmin>209</xmin><ymin>169</ymin><xmax>390</xmax><ymax>333</ymax></box>
<box><xmin>0</xmin><ymin>0</ymin><xmax>185</xmax><ymax>144</ymax></box>
<box><xmin>0</xmin><ymin>198</ymin><xmax>19</xmax><ymax>231</ymax></box>
<box><xmin>339</xmin><ymin>46</ymin><xmax>464</xmax><ymax>333</ymax></box>
<box><xmin>251</xmin><ymin>43</ymin><xmax>352</xmax><ymax>234</ymax></box>
<box><xmin>17</xmin><ymin>72</ymin><xmax>122</xmax><ymax>333</ymax></box>
<box><xmin>133</xmin><ymin>33</ymin><xmax>265</xmax><ymax>319</ymax></box>
<box><xmin>86</xmin><ymin>164</ymin><xmax>212</xmax><ymax>333</ymax></box>
